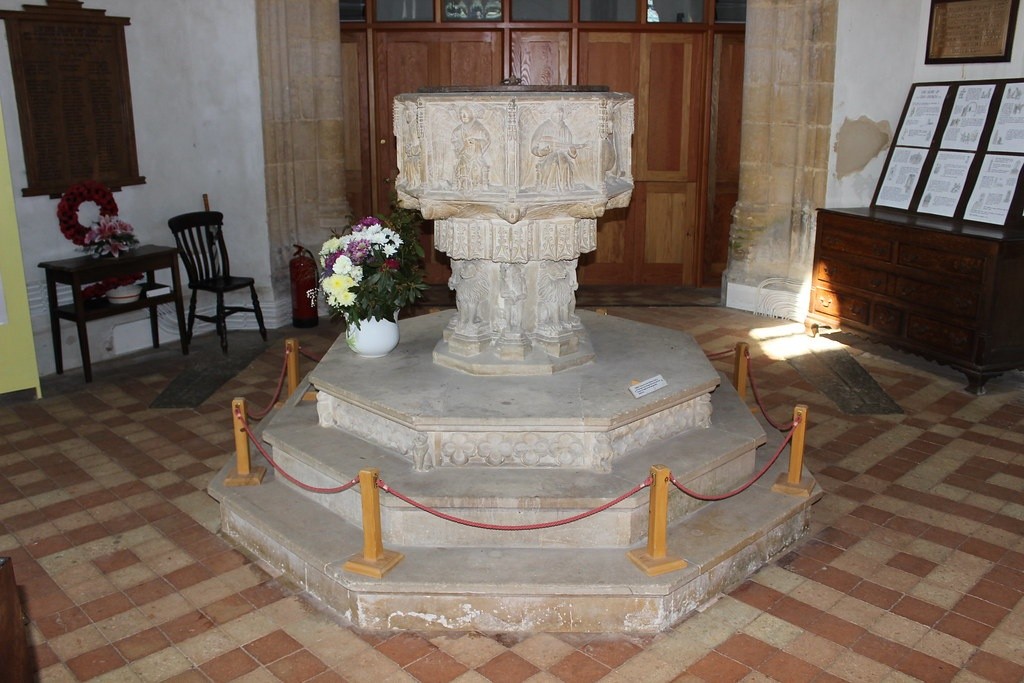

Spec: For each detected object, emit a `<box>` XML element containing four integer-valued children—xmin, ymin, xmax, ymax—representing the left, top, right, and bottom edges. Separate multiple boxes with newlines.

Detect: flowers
<box><xmin>84</xmin><ymin>213</ymin><xmax>139</xmax><ymax>260</ymax></box>
<box><xmin>303</xmin><ymin>187</ymin><xmax>434</xmax><ymax>334</ymax></box>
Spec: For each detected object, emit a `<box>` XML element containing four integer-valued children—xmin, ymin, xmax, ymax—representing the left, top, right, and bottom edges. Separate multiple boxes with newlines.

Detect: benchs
<box><xmin>0</xmin><ymin>557</ymin><xmax>34</xmax><ymax>683</ymax></box>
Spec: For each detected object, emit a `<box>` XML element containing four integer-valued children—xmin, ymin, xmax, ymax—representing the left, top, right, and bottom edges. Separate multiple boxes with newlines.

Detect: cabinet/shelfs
<box><xmin>38</xmin><ymin>243</ymin><xmax>190</xmax><ymax>383</ymax></box>
<box><xmin>0</xmin><ymin>104</ymin><xmax>44</xmax><ymax>401</ymax></box>
<box><xmin>575</xmin><ymin>29</ymin><xmax>709</xmax><ymax>181</ymax></box>
<box><xmin>577</xmin><ymin>181</ymin><xmax>705</xmax><ymax>290</ymax></box>
<box><xmin>804</xmin><ymin>205</ymin><xmax>1024</xmax><ymax>396</ymax></box>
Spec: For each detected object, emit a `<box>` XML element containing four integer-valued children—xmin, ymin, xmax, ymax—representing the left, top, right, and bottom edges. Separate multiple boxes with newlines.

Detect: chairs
<box><xmin>167</xmin><ymin>211</ymin><xmax>269</xmax><ymax>353</ymax></box>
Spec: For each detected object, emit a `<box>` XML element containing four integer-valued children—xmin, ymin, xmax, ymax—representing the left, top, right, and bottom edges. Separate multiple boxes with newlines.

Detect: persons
<box><xmin>530</xmin><ymin>109</ymin><xmax>577</xmax><ymax>191</ymax></box>
<box><xmin>403</xmin><ymin>110</ymin><xmax>421</xmax><ymax>188</ymax></box>
<box><xmin>452</xmin><ymin>108</ymin><xmax>492</xmax><ymax>190</ymax></box>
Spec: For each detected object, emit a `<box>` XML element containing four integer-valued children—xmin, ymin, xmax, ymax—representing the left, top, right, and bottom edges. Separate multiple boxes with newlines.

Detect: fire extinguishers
<box><xmin>289</xmin><ymin>244</ymin><xmax>320</xmax><ymax>328</ymax></box>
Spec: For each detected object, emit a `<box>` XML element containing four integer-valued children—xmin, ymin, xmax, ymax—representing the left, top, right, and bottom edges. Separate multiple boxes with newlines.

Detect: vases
<box><xmin>103</xmin><ymin>247</ymin><xmax>127</xmax><ymax>258</ymax></box>
<box><xmin>343</xmin><ymin>307</ymin><xmax>401</xmax><ymax>359</ymax></box>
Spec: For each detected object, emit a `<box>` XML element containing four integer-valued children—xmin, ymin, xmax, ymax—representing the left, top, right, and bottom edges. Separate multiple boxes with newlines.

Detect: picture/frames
<box><xmin>924</xmin><ymin>0</ymin><xmax>1021</xmax><ymax>64</ymax></box>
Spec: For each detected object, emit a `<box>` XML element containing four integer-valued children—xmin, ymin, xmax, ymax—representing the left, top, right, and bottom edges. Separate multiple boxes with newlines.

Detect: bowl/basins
<box><xmin>106</xmin><ymin>285</ymin><xmax>142</xmax><ymax>304</ymax></box>
